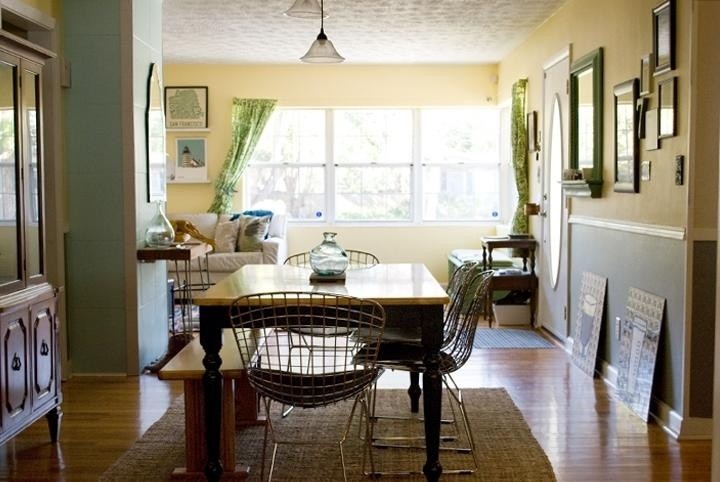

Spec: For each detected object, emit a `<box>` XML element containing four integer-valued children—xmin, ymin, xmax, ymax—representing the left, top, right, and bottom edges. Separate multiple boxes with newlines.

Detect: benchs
<box><xmin>158</xmin><ymin>321</ymin><xmax>258</xmax><ymax>480</ymax></box>
<box><xmin>448</xmin><ymin>250</ymin><xmax>514</xmax><ymax>320</ymax></box>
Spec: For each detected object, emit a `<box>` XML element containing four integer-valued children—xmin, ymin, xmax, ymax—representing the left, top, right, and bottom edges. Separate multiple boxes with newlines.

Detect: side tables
<box><xmin>480</xmin><ymin>232</ymin><xmax>538</xmax><ymax>329</ymax></box>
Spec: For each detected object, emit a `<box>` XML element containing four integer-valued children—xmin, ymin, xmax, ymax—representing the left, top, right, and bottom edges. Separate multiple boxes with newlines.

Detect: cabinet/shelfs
<box><xmin>136</xmin><ymin>240</ymin><xmax>214</xmax><ymax>349</ymax></box>
<box><xmin>0</xmin><ymin>28</ymin><xmax>77</xmax><ymax>459</ymax></box>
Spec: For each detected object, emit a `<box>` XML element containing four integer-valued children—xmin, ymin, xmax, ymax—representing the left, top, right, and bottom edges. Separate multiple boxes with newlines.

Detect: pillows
<box><xmin>213</xmin><ymin>215</ymin><xmax>271</xmax><ymax>253</ymax></box>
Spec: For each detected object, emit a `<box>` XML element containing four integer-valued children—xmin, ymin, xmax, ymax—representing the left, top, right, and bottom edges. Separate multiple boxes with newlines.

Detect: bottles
<box><xmin>143</xmin><ymin>200</ymin><xmax>175</xmax><ymax>247</ymax></box>
<box><xmin>309</xmin><ymin>232</ymin><xmax>349</xmax><ymax>274</ymax></box>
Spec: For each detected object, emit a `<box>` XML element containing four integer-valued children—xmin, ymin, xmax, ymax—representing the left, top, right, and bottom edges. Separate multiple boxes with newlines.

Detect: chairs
<box><xmin>362</xmin><ymin>260</ymin><xmax>494</xmax><ymax>443</ymax></box>
<box><xmin>278</xmin><ymin>246</ymin><xmax>387</xmax><ymax>419</ymax></box>
<box><xmin>367</xmin><ymin>269</ymin><xmax>498</xmax><ymax>477</ymax></box>
<box><xmin>230</xmin><ymin>291</ymin><xmax>386</xmax><ymax>482</ymax></box>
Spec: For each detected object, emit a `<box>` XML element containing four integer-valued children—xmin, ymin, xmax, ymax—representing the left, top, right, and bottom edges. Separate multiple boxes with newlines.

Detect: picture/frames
<box><xmin>165</xmin><ymin>86</ymin><xmax>212</xmax><ymax>131</ymax></box>
<box><xmin>145</xmin><ymin>63</ymin><xmax>169</xmax><ymax>204</ymax></box>
<box><xmin>563</xmin><ymin>48</ymin><xmax>604</xmax><ymax>200</ymax></box>
<box><xmin>612</xmin><ymin>76</ymin><xmax>643</xmax><ymax>194</ymax></box>
<box><xmin>630</xmin><ymin>3</ymin><xmax>679</xmax><ymax>153</ymax></box>
<box><xmin>527</xmin><ymin>111</ymin><xmax>537</xmax><ymax>154</ymax></box>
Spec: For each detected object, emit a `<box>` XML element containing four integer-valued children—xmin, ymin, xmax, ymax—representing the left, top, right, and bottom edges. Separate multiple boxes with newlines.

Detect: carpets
<box><xmin>456</xmin><ymin>328</ymin><xmax>556</xmax><ymax>349</ymax></box>
<box><xmin>92</xmin><ymin>386</ymin><xmax>557</xmax><ymax>482</ymax></box>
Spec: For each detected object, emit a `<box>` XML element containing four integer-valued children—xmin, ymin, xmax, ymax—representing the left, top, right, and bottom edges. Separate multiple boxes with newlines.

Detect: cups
<box><xmin>524</xmin><ymin>203</ymin><xmax>540</xmax><ymax>215</ymax></box>
<box><xmin>563</xmin><ymin>168</ymin><xmax>594</xmax><ymax>179</ymax></box>
<box><xmin>624</xmin><ymin>317</ymin><xmax>648</xmax><ymax>398</ymax></box>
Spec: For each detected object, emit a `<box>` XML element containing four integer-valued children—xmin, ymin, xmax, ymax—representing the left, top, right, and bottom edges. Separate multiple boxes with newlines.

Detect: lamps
<box><xmin>284</xmin><ymin>1</ymin><xmax>330</xmax><ymax>19</ymax></box>
<box><xmin>299</xmin><ymin>3</ymin><xmax>346</xmax><ymax>65</ymax></box>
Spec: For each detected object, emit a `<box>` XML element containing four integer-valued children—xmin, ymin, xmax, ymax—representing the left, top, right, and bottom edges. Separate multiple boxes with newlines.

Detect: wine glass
<box><xmin>574</xmin><ymin>293</ymin><xmax>598</xmax><ymax>361</ymax></box>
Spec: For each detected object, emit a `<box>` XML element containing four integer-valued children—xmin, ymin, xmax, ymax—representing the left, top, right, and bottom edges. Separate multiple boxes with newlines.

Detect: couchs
<box><xmin>168</xmin><ymin>210</ymin><xmax>290</xmax><ymax>301</ymax></box>
